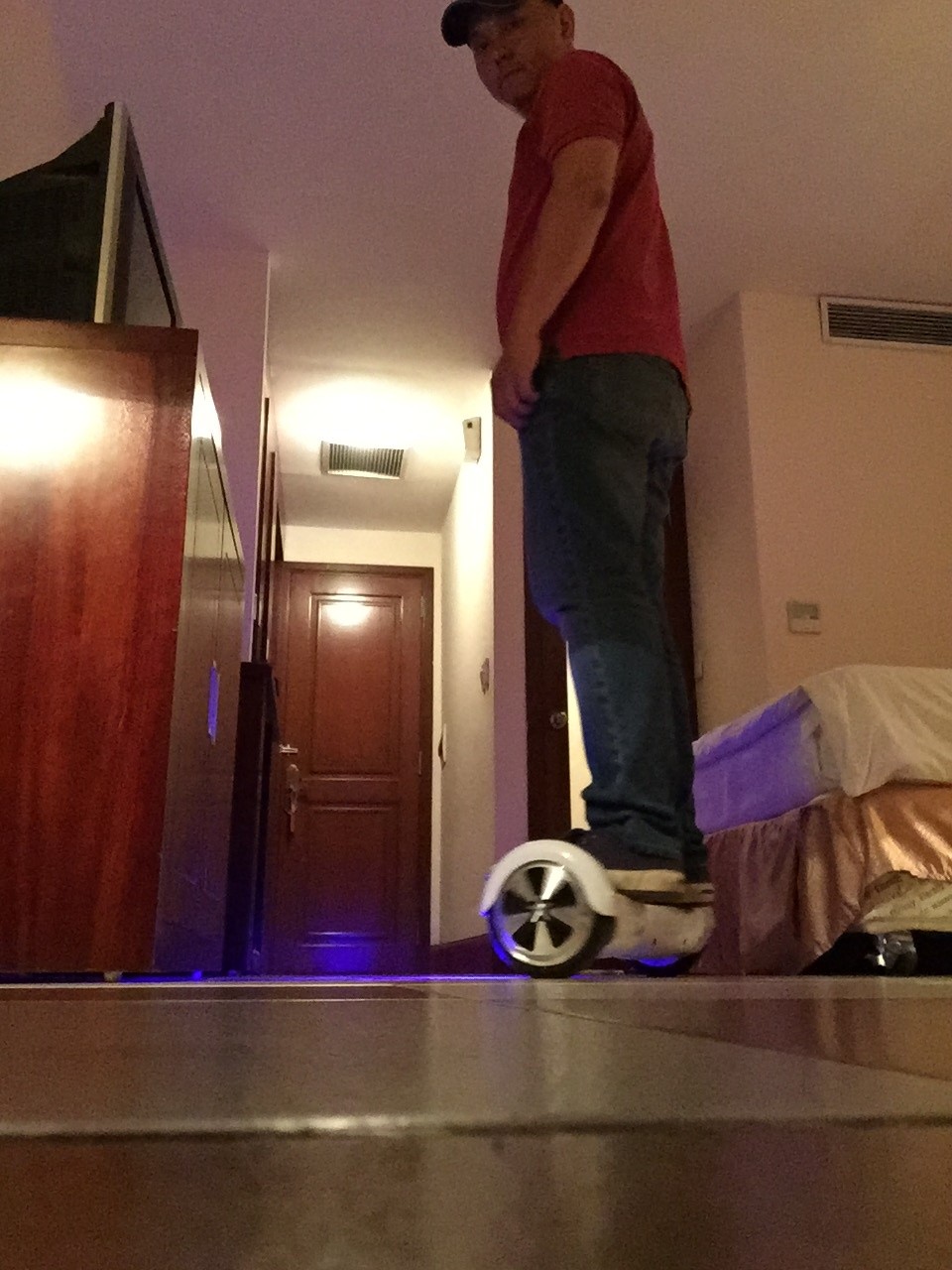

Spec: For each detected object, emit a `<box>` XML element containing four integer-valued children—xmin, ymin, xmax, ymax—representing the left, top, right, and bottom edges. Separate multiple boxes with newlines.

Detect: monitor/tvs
<box><xmin>0</xmin><ymin>101</ymin><xmax>183</xmax><ymax>332</ymax></box>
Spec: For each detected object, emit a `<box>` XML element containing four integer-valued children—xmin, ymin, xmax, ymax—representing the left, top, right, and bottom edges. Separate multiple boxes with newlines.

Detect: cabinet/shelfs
<box><xmin>0</xmin><ymin>317</ymin><xmax>247</xmax><ymax>982</ymax></box>
<box><xmin>229</xmin><ymin>397</ymin><xmax>283</xmax><ymax>976</ymax></box>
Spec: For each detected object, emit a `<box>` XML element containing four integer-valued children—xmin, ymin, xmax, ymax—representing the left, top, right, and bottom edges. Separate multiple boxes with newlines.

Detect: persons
<box><xmin>440</xmin><ymin>0</ymin><xmax>715</xmax><ymax>905</ymax></box>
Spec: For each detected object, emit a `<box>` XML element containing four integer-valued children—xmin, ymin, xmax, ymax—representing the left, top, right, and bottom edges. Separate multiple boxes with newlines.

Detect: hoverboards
<box><xmin>481</xmin><ymin>824</ymin><xmax>715</xmax><ymax>983</ymax></box>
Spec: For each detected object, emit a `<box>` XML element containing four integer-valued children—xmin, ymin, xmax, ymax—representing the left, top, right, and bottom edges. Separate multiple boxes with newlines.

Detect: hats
<box><xmin>441</xmin><ymin>0</ymin><xmax>522</xmax><ymax>48</ymax></box>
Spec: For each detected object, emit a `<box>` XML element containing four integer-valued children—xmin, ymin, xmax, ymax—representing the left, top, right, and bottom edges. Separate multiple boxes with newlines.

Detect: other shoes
<box><xmin>624</xmin><ymin>879</ymin><xmax>717</xmax><ymax>906</ymax></box>
<box><xmin>566</xmin><ymin>828</ymin><xmax>688</xmax><ymax>895</ymax></box>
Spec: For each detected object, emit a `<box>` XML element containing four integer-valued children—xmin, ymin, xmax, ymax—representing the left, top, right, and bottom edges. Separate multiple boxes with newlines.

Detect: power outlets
<box><xmin>787</xmin><ymin>603</ymin><xmax>821</xmax><ymax>634</ymax></box>
<box><xmin>480</xmin><ymin>658</ymin><xmax>489</xmax><ymax>692</ymax></box>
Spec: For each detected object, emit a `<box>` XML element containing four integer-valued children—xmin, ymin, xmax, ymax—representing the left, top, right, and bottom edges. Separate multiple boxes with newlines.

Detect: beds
<box><xmin>691</xmin><ymin>665</ymin><xmax>952</xmax><ymax>976</ymax></box>
